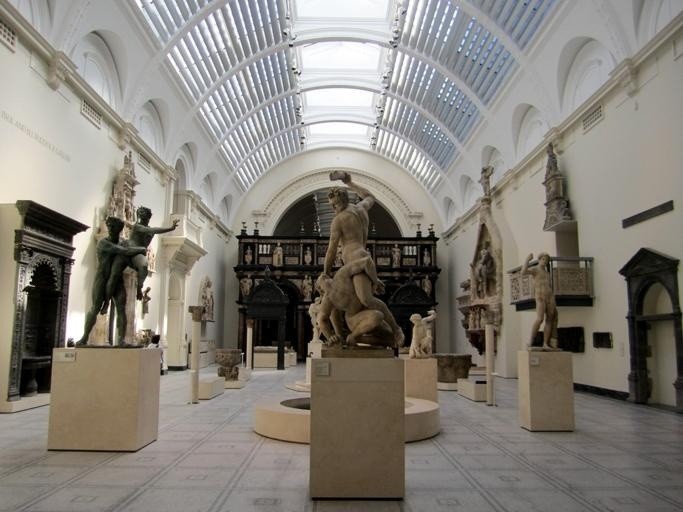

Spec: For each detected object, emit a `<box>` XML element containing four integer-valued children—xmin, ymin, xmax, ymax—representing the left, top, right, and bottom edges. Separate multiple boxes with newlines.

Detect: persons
<box><xmin>149</xmin><ymin>335</ymin><xmax>164</xmax><ymax>369</ymax></box>
<box><xmin>523</xmin><ymin>252</ymin><xmax>556</xmax><ymax>351</ymax></box>
<box><xmin>273</xmin><ymin>241</ymin><xmax>283</xmax><ymax>265</ymax></box>
<box><xmin>304</xmin><ymin>246</ymin><xmax>312</xmax><ymax>264</ymax></box>
<box><xmin>100</xmin><ymin>206</ymin><xmax>181</xmax><ymax>316</ymax></box>
<box><xmin>322</xmin><ymin>169</ymin><xmax>406</xmax><ymax>347</ymax></box>
<box><xmin>409</xmin><ymin>310</ymin><xmax>436</xmax><ymax>358</ymax></box>
<box><xmin>244</xmin><ymin>245</ymin><xmax>253</xmax><ymax>265</ymax></box>
<box><xmin>422</xmin><ymin>247</ymin><xmax>430</xmax><ymax>265</ymax></box>
<box><xmin>309</xmin><ymin>297</ymin><xmax>322</xmax><ymax>342</ymax></box>
<box><xmin>314</xmin><ymin>255</ymin><xmax>393</xmax><ymax>349</ymax></box>
<box><xmin>76</xmin><ymin>216</ymin><xmax>146</xmax><ymax>347</ymax></box>
<box><xmin>424</xmin><ymin>274</ymin><xmax>432</xmax><ymax>296</ymax></box>
